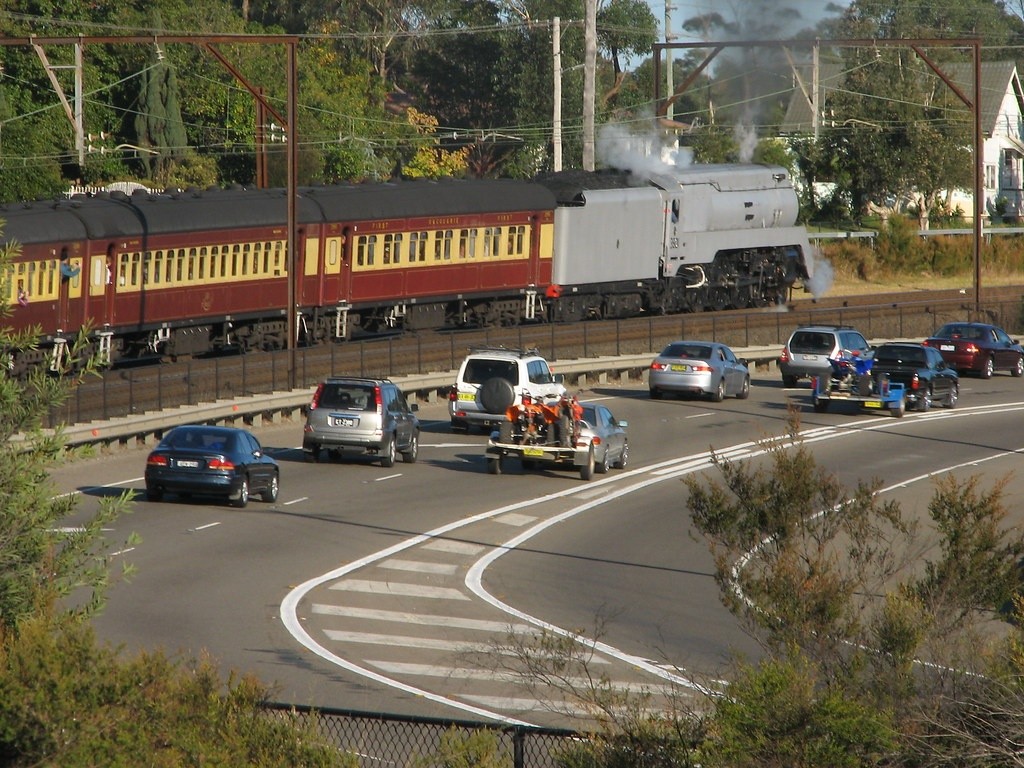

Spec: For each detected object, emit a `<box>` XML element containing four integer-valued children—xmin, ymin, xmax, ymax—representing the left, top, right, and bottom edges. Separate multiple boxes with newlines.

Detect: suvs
<box><xmin>302</xmin><ymin>376</ymin><xmax>422</xmax><ymax>468</ymax></box>
<box><xmin>868</xmin><ymin>340</ymin><xmax>961</xmax><ymax>413</ymax></box>
<box><xmin>776</xmin><ymin>323</ymin><xmax>877</xmax><ymax>387</ymax></box>
<box><xmin>447</xmin><ymin>344</ymin><xmax>567</xmax><ymax>432</ymax></box>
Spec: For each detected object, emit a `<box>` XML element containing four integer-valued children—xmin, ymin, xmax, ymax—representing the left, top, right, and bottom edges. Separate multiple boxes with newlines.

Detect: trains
<box><xmin>0</xmin><ymin>160</ymin><xmax>814</xmax><ymax>383</ymax></box>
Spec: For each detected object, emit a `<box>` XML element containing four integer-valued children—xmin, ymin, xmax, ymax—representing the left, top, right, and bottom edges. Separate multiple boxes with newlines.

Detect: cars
<box><xmin>145</xmin><ymin>424</ymin><xmax>281</xmax><ymax>509</ymax></box>
<box><xmin>648</xmin><ymin>339</ymin><xmax>750</xmax><ymax>403</ymax></box>
<box><xmin>544</xmin><ymin>400</ymin><xmax>630</xmax><ymax>477</ymax></box>
<box><xmin>922</xmin><ymin>321</ymin><xmax>1024</xmax><ymax>380</ymax></box>
